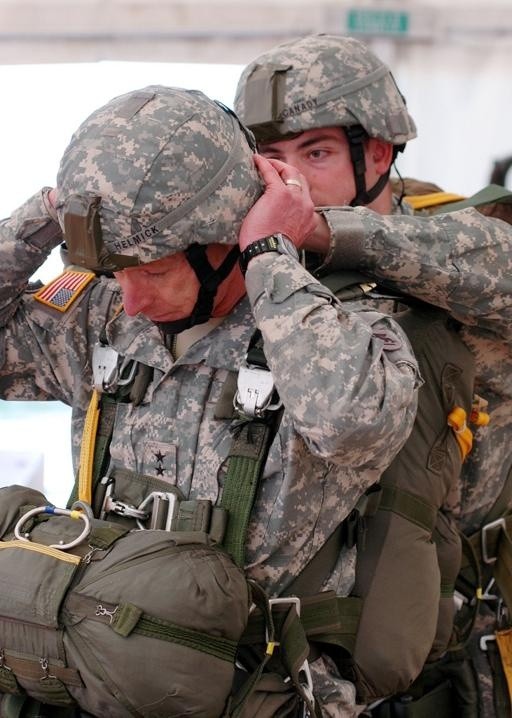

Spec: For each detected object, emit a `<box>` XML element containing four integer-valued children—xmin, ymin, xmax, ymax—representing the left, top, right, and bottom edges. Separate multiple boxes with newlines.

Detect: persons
<box><xmin>2</xmin><ymin>83</ymin><xmax>427</xmax><ymax>717</ymax></box>
<box><xmin>226</xmin><ymin>29</ymin><xmax>511</xmax><ymax>715</ymax></box>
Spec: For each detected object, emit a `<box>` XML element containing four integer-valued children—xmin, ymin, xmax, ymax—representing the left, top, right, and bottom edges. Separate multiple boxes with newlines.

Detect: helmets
<box><xmin>56</xmin><ymin>86</ymin><xmax>263</xmax><ymax>271</ymax></box>
<box><xmin>234</xmin><ymin>35</ymin><xmax>416</xmax><ymax>144</ymax></box>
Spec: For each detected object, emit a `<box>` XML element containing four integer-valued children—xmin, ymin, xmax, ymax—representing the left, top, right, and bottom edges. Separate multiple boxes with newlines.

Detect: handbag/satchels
<box><xmin>0</xmin><ymin>485</ymin><xmax>248</xmax><ymax>718</ymax></box>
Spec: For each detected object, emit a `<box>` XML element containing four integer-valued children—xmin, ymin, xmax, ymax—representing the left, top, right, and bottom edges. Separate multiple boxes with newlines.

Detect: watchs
<box><xmin>237</xmin><ymin>230</ymin><xmax>301</xmax><ymax>278</ymax></box>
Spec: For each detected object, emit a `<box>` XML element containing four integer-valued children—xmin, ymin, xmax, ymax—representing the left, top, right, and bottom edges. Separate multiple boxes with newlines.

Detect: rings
<box><xmin>284</xmin><ymin>179</ymin><xmax>303</xmax><ymax>190</ymax></box>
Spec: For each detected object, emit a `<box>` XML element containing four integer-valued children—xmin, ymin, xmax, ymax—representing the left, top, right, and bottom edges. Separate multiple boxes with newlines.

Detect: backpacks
<box><xmin>214</xmin><ymin>276</ymin><xmax>475</xmax><ymax>698</ymax></box>
